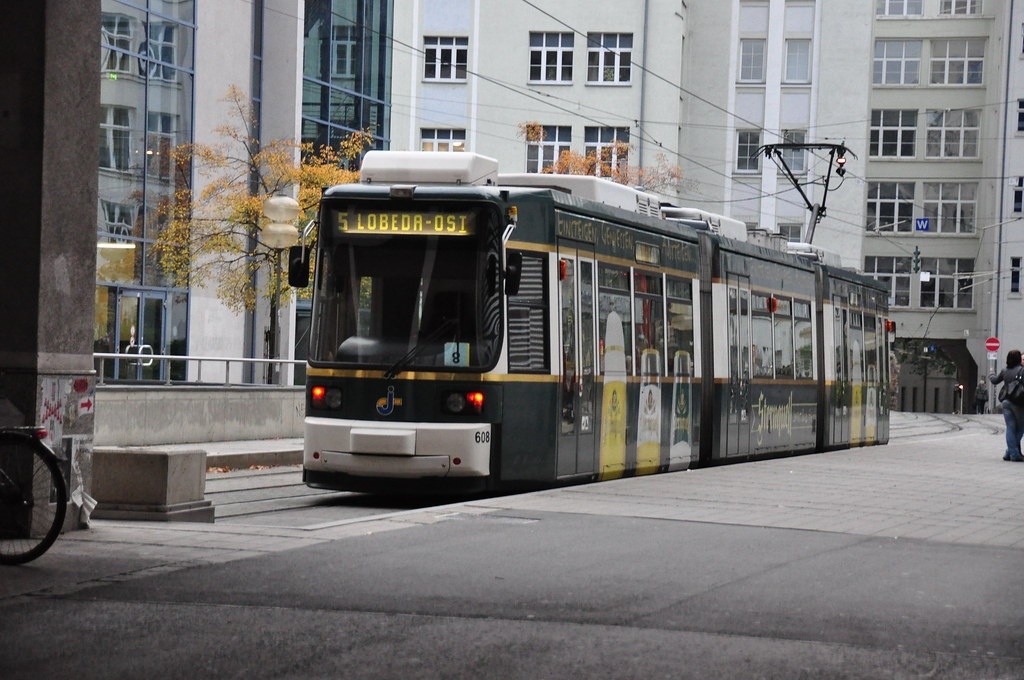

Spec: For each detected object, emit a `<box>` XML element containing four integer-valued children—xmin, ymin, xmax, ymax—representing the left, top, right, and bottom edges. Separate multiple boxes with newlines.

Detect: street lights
<box><xmin>259</xmin><ymin>193</ymin><xmax>303</xmax><ymax>387</ymax></box>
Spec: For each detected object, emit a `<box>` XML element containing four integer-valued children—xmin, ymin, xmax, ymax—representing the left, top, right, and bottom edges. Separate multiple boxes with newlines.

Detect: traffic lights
<box><xmin>912</xmin><ymin>245</ymin><xmax>922</xmax><ymax>274</ymax></box>
<box><xmin>836</xmin><ymin>147</ymin><xmax>847</xmax><ymax>178</ymax></box>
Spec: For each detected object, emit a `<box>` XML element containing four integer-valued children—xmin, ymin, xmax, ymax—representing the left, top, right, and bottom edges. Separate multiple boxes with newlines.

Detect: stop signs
<box><xmin>985</xmin><ymin>337</ymin><xmax>1000</xmax><ymax>352</ymax></box>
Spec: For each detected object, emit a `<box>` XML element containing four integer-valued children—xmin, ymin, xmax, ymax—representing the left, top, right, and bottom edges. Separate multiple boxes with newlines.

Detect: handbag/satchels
<box><xmin>1006</xmin><ymin>367</ymin><xmax>1024</xmax><ymax>406</ymax></box>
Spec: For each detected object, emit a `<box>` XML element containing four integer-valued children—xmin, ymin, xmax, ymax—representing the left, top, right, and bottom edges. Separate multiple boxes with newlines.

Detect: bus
<box><xmin>286</xmin><ymin>140</ymin><xmax>896</xmax><ymax>495</ymax></box>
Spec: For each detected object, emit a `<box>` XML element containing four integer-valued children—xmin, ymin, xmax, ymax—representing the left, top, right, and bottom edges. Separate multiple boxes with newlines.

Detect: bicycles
<box><xmin>1</xmin><ymin>425</ymin><xmax>69</xmax><ymax>567</ymax></box>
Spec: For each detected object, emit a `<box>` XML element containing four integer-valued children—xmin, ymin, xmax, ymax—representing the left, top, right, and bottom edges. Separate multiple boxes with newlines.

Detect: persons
<box><xmin>975</xmin><ymin>380</ymin><xmax>988</xmax><ymax>414</ymax></box>
<box><xmin>988</xmin><ymin>350</ymin><xmax>1024</xmax><ymax>462</ymax></box>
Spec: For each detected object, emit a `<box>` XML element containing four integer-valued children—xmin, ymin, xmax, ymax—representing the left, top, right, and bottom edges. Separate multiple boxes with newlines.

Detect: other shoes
<box><xmin>1003</xmin><ymin>449</ymin><xmax>1011</xmax><ymax>461</ymax></box>
<box><xmin>1009</xmin><ymin>453</ymin><xmax>1024</xmax><ymax>462</ymax></box>
